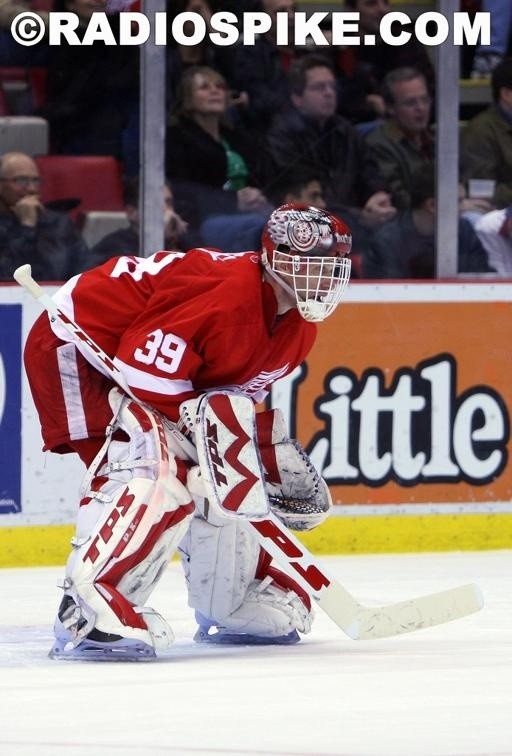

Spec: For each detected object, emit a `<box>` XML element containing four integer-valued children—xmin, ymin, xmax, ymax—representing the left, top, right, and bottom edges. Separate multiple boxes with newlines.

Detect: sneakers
<box><xmin>58</xmin><ymin>597</ymin><xmax>122</xmax><ymax>642</ymax></box>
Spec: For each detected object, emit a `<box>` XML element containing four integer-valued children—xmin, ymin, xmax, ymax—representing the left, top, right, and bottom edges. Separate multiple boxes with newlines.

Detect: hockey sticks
<box><xmin>13</xmin><ymin>264</ymin><xmax>485</xmax><ymax>639</ymax></box>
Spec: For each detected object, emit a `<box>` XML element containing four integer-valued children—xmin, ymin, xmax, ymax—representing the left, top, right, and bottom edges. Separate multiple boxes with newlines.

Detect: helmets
<box><xmin>260</xmin><ymin>202</ymin><xmax>354</xmax><ymax>323</ymax></box>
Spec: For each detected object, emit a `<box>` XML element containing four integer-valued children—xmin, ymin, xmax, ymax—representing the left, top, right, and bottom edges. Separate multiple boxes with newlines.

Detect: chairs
<box><xmin>5</xmin><ymin>111</ymin><xmax>130</xmax><ymax>244</ymax></box>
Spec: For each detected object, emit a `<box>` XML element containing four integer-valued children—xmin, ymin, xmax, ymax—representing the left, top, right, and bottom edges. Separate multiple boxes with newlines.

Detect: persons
<box><xmin>25</xmin><ymin>201</ymin><xmax>356</xmax><ymax>653</ymax></box>
<box><xmin>0</xmin><ymin>1</ymin><xmax>512</xmax><ymax>283</ymax></box>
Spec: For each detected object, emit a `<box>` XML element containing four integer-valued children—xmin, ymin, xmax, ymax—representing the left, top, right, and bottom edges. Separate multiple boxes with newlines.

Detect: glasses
<box><xmin>2</xmin><ymin>176</ymin><xmax>42</xmax><ymax>185</ymax></box>
